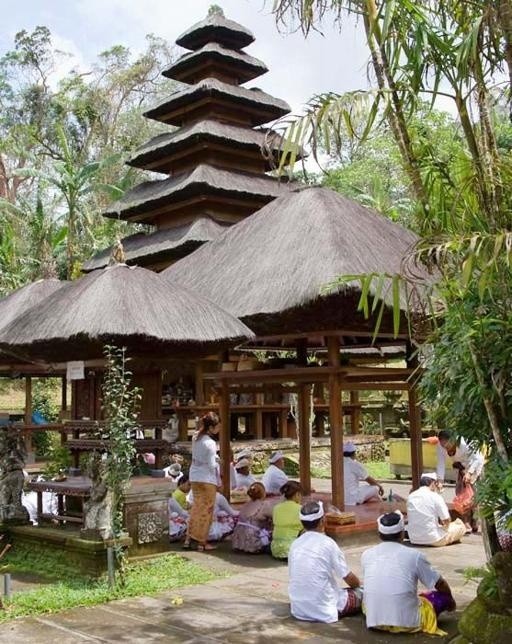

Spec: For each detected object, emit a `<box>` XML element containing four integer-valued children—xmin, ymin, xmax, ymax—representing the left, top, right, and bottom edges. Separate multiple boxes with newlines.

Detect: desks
<box><xmin>389</xmin><ymin>438</ymin><xmax>442</xmax><ymax>480</ymax></box>
<box><xmin>28</xmin><ymin>477</ymin><xmax>94</xmax><ymax>527</ymax></box>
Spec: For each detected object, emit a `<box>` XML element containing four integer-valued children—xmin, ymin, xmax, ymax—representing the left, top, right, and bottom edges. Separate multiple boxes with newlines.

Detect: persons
<box><xmin>406</xmin><ymin>472</ymin><xmax>467</xmax><ymax>547</ymax></box>
<box><xmin>233</xmin><ymin>482</ymin><xmax>276</xmax><ymax>555</ymax></box>
<box><xmin>343</xmin><ymin>441</ymin><xmax>384</xmax><ymax>506</ymax></box>
<box><xmin>183</xmin><ymin>413</ymin><xmax>221</xmax><ymax>549</ymax></box>
<box><xmin>264</xmin><ymin>450</ymin><xmax>289</xmax><ymax>496</ymax></box>
<box><xmin>234</xmin><ymin>449</ymin><xmax>250</xmax><ymax>472</ymax></box>
<box><xmin>235</xmin><ymin>460</ymin><xmax>256</xmax><ymax>491</ymax></box>
<box><xmin>207</xmin><ymin>488</ymin><xmax>239</xmax><ymax>541</ymax></box>
<box><xmin>287</xmin><ymin>502</ymin><xmax>364</xmax><ymax>622</ymax></box>
<box><xmin>272</xmin><ymin>480</ymin><xmax>309</xmax><ymax>561</ymax></box>
<box><xmin>173</xmin><ymin>475</ymin><xmax>194</xmax><ymax>509</ymax></box>
<box><xmin>168</xmin><ymin>496</ymin><xmax>190</xmax><ymax>541</ymax></box>
<box><xmin>163</xmin><ymin>463</ymin><xmax>184</xmax><ymax>484</ymax></box>
<box><xmin>169</xmin><ymin>462</ymin><xmax>187</xmax><ymax>484</ymax></box>
<box><xmin>437</xmin><ymin>428</ymin><xmax>484</xmax><ymax>533</ymax></box>
<box><xmin>361</xmin><ymin>510</ymin><xmax>456</xmax><ymax>639</ymax></box>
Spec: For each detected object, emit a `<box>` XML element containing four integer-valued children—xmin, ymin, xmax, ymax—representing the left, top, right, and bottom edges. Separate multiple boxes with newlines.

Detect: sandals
<box><xmin>183</xmin><ymin>543</ymin><xmax>192</xmax><ymax>550</ymax></box>
<box><xmin>197</xmin><ymin>543</ymin><xmax>217</xmax><ymax>551</ymax></box>
